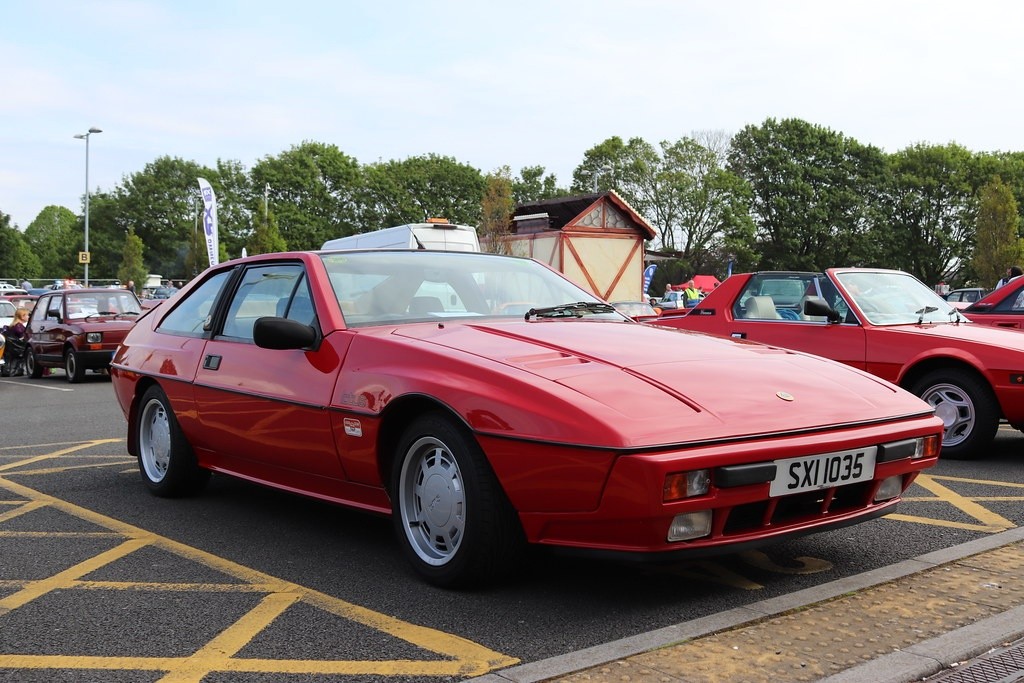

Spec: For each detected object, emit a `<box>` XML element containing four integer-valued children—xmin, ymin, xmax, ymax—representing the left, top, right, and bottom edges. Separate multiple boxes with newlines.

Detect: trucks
<box><xmin>319</xmin><ymin>218</ymin><xmax>491</xmax><ymax>313</ymax></box>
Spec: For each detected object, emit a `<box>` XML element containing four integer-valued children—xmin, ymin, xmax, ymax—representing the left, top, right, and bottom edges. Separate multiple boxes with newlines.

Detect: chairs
<box><xmin>275</xmin><ymin>297</ymin><xmax>316</xmax><ymax>324</ymax></box>
<box><xmin>743</xmin><ymin>296</ymin><xmax>784</xmax><ymax>321</ymax></box>
<box><xmin>799</xmin><ymin>295</ymin><xmax>831</xmax><ymax>323</ymax></box>
<box><xmin>409</xmin><ymin>297</ymin><xmax>447</xmax><ymax>316</ymax></box>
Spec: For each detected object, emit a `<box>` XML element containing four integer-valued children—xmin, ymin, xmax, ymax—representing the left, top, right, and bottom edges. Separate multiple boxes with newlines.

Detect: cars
<box><xmin>0</xmin><ymin>284</ymin><xmax>179</xmax><ymax>365</ymax></box>
<box><xmin>631</xmin><ymin>267</ymin><xmax>1023</xmax><ymax>461</ymax></box>
<box><xmin>111</xmin><ymin>251</ymin><xmax>944</xmax><ymax>584</ymax></box>
<box><xmin>610</xmin><ymin>291</ymin><xmax>706</xmax><ymax>322</ymax></box>
<box><xmin>24</xmin><ymin>289</ymin><xmax>144</xmax><ymax>384</ymax></box>
<box><xmin>947</xmin><ymin>288</ymin><xmax>995</xmax><ymax>310</ymax></box>
<box><xmin>959</xmin><ymin>276</ymin><xmax>1024</xmax><ymax>329</ymax></box>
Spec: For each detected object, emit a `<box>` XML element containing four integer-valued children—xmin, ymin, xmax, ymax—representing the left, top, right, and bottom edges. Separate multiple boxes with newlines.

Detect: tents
<box><xmin>671</xmin><ymin>275</ymin><xmax>722</xmax><ymax>293</ymax></box>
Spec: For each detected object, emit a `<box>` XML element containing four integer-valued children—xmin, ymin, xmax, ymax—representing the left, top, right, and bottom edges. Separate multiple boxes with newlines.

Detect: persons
<box><xmin>714</xmin><ymin>282</ymin><xmax>719</xmax><ymax>287</ymax></box>
<box><xmin>22</xmin><ymin>278</ymin><xmax>33</xmax><ymax>292</ymax></box>
<box><xmin>995</xmin><ymin>267</ymin><xmax>1024</xmax><ymax>307</ymax></box>
<box><xmin>683</xmin><ymin>281</ymin><xmax>700</xmax><ymax>309</ymax></box>
<box><xmin>661</xmin><ymin>284</ymin><xmax>673</xmax><ymax>301</ymax></box>
<box><xmin>178</xmin><ymin>282</ymin><xmax>183</xmax><ymax>289</ymax></box>
<box><xmin>697</xmin><ymin>286</ymin><xmax>709</xmax><ymax>297</ymax></box>
<box><xmin>128</xmin><ymin>281</ymin><xmax>135</xmax><ymax>294</ymax></box>
<box><xmin>649</xmin><ymin>299</ymin><xmax>658</xmax><ymax>308</ymax></box>
<box><xmin>10</xmin><ymin>307</ymin><xmax>51</xmax><ymax>375</ymax></box>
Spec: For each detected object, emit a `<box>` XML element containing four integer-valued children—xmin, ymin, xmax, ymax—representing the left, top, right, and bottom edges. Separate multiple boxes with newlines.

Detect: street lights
<box><xmin>265</xmin><ymin>182</ymin><xmax>271</xmax><ymax>217</ymax></box>
<box><xmin>193</xmin><ymin>194</ymin><xmax>203</xmax><ymax>277</ymax></box>
<box><xmin>73</xmin><ymin>126</ymin><xmax>103</xmax><ymax>289</ymax></box>
